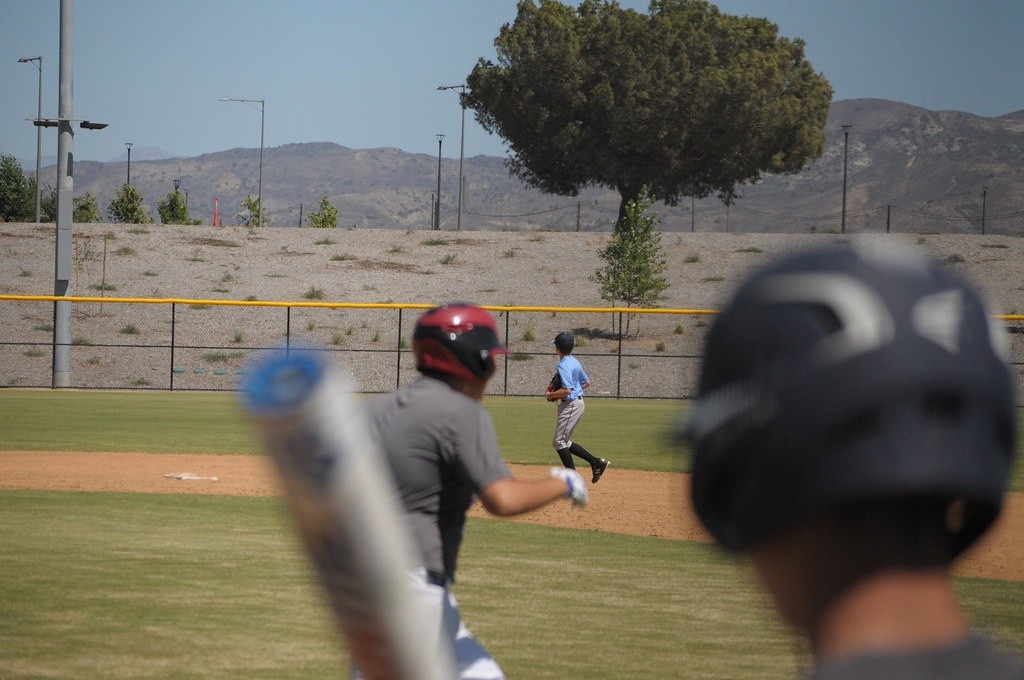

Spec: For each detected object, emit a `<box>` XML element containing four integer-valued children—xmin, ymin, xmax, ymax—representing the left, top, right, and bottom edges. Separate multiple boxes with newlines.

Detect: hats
<box><xmin>553</xmin><ymin>332</ymin><xmax>574</xmax><ymax>345</ymax></box>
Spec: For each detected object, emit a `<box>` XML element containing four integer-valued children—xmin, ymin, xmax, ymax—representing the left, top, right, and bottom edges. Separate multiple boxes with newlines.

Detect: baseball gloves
<box><xmin>544</xmin><ymin>374</ymin><xmax>562</xmax><ymax>402</ymax></box>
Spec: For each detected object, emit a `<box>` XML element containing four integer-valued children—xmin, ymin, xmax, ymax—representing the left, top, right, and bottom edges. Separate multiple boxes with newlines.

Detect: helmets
<box><xmin>412</xmin><ymin>302</ymin><xmax>509</xmax><ymax>398</ymax></box>
<box><xmin>684</xmin><ymin>231</ymin><xmax>1015</xmax><ymax>573</ymax></box>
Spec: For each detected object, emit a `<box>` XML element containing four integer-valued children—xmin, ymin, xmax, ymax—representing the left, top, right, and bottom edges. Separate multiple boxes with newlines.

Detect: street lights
<box><xmin>980</xmin><ymin>184</ymin><xmax>989</xmax><ymax>240</ymax></box>
<box><xmin>841</xmin><ymin>124</ymin><xmax>853</xmax><ymax>235</ymax></box>
<box><xmin>124</xmin><ymin>142</ymin><xmax>133</xmax><ymax>184</ymax></box>
<box><xmin>18</xmin><ymin>55</ymin><xmax>44</xmax><ymax>226</ymax></box>
<box><xmin>435</xmin><ymin>132</ymin><xmax>446</xmax><ymax>232</ymax></box>
<box><xmin>31</xmin><ymin>119</ymin><xmax>110</xmax><ymax>390</ymax></box>
<box><xmin>218</xmin><ymin>97</ymin><xmax>265</xmax><ymax>228</ymax></box>
<box><xmin>437</xmin><ymin>83</ymin><xmax>467</xmax><ymax>227</ymax></box>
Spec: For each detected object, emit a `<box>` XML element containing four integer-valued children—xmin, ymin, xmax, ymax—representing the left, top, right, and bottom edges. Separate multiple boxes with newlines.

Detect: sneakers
<box><xmin>591</xmin><ymin>458</ymin><xmax>610</xmax><ymax>483</ymax></box>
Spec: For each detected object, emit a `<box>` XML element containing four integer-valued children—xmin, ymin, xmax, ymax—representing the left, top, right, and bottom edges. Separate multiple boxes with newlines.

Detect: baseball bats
<box><xmin>237</xmin><ymin>340</ymin><xmax>464</xmax><ymax>680</ymax></box>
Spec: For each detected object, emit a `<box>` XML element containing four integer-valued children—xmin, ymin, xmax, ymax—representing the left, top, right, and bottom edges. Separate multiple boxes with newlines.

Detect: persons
<box><xmin>352</xmin><ymin>303</ymin><xmax>589</xmax><ymax>679</ymax></box>
<box><xmin>652</xmin><ymin>232</ymin><xmax>1022</xmax><ymax>680</ymax></box>
<box><xmin>544</xmin><ymin>332</ymin><xmax>607</xmax><ymax>483</ymax></box>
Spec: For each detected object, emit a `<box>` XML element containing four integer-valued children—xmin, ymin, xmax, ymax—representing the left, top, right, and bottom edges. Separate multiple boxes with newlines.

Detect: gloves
<box><xmin>550</xmin><ymin>467</ymin><xmax>589</xmax><ymax>512</ymax></box>
